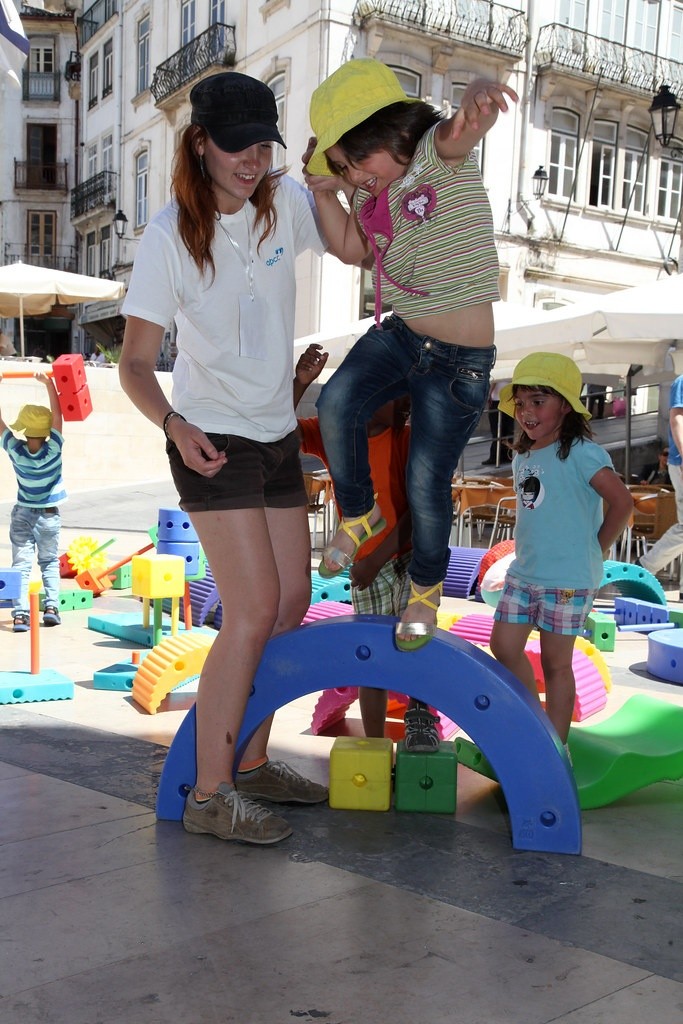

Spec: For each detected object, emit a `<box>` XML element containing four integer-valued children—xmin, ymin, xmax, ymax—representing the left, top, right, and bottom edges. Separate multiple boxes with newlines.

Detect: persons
<box><xmin>635</xmin><ymin>372</ymin><xmax>682</xmax><ymax>603</ymax></box>
<box><xmin>636</xmin><ymin>447</ymin><xmax>672</xmax><ymax>486</ymax></box>
<box><xmin>291</xmin><ymin>344</ymin><xmax>441</xmax><ymax>790</ymax></box>
<box><xmin>490</xmin><ymin>352</ymin><xmax>635</xmax><ymax>769</ymax></box>
<box><xmin>118</xmin><ymin>72</ymin><xmax>381</xmax><ymax>844</ymax></box>
<box><xmin>0</xmin><ymin>370</ymin><xmax>65</xmax><ymax>632</ymax></box>
<box><xmin>301</xmin><ymin>55</ymin><xmax>518</xmax><ymax>651</ymax></box>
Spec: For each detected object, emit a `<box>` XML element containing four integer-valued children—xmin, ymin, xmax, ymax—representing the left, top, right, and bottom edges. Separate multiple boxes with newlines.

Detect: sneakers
<box><xmin>403</xmin><ymin>709</ymin><xmax>441</xmax><ymax>752</ymax></box>
<box><xmin>181</xmin><ymin>760</ymin><xmax>330</xmax><ymax>844</ymax></box>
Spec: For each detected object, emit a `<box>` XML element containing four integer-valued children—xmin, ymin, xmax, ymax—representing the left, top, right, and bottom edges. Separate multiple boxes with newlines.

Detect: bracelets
<box><xmin>163</xmin><ymin>412</ymin><xmax>186</xmax><ymax>444</ymax></box>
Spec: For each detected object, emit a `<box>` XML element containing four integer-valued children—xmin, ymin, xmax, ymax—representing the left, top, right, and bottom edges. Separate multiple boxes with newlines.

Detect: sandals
<box><xmin>318</xmin><ymin>492</ymin><xmax>386</xmax><ymax>578</ymax></box>
<box><xmin>43</xmin><ymin>605</ymin><xmax>61</xmax><ymax>625</ymax></box>
<box><xmin>395</xmin><ymin>578</ymin><xmax>443</xmax><ymax>652</ymax></box>
<box><xmin>11</xmin><ymin>611</ymin><xmax>31</xmax><ymax>631</ymax></box>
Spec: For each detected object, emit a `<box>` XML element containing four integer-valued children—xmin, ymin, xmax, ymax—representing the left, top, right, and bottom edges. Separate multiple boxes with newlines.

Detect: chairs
<box><xmin>304</xmin><ymin>466</ymin><xmax>683</xmax><ymax>587</ymax></box>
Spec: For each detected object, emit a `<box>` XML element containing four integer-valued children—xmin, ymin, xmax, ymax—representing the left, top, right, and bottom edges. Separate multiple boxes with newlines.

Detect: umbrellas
<box><xmin>493</xmin><ymin>273</ymin><xmax>683</xmax><ymax>368</ymax></box>
<box><xmin>292</xmin><ymin>280</ymin><xmax>622</xmax><ymax>388</ymax></box>
<box><xmin>0</xmin><ymin>259</ymin><xmax>125</xmax><ymax>357</ymax></box>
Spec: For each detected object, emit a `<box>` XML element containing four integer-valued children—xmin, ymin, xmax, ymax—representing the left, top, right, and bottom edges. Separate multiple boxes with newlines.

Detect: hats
<box><xmin>497</xmin><ymin>352</ymin><xmax>592</xmax><ymax>422</ymax></box>
<box><xmin>189</xmin><ymin>72</ymin><xmax>287</xmax><ymax>153</ymax></box>
<box><xmin>305</xmin><ymin>59</ymin><xmax>424</xmax><ymax>176</ymax></box>
<box><xmin>9</xmin><ymin>404</ymin><xmax>52</xmax><ymax>437</ymax></box>
